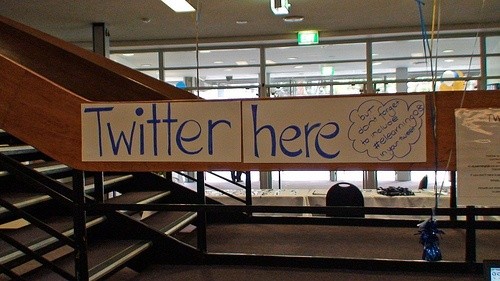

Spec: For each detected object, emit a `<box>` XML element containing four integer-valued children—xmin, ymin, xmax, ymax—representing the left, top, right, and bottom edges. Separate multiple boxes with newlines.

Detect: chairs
<box><xmin>325</xmin><ymin>181</ymin><xmax>366</xmax><ymax>218</ymax></box>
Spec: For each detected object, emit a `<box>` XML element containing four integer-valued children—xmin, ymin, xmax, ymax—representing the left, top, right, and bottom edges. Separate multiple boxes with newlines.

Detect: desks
<box><xmin>205</xmin><ymin>187</ymin><xmax>452</xmax><ymax>220</ymax></box>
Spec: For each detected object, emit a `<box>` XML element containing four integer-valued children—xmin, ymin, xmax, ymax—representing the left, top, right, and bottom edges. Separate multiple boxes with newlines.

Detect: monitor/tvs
<box><xmin>482</xmin><ymin>259</ymin><xmax>500</xmax><ymax>281</ymax></box>
